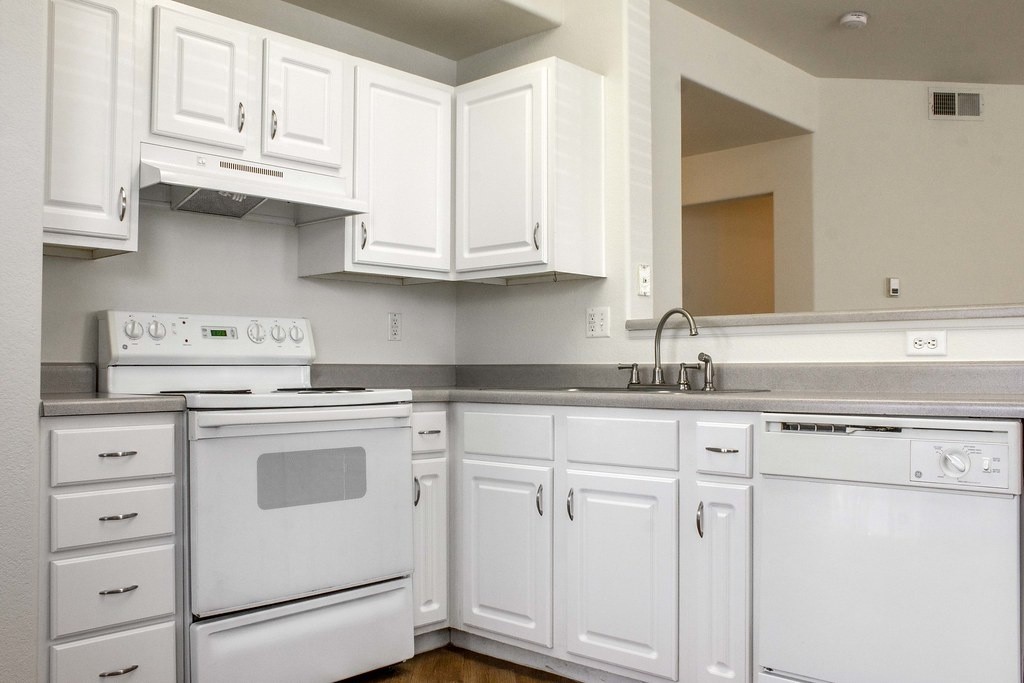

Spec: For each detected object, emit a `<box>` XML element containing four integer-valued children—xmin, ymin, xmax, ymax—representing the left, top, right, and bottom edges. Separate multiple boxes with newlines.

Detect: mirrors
<box><xmin>649</xmin><ymin>0</ymin><xmax>1024</xmax><ymax>330</ymax></box>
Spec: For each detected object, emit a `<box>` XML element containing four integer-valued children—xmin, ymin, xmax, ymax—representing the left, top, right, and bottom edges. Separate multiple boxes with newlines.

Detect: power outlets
<box><xmin>388</xmin><ymin>313</ymin><xmax>401</xmax><ymax>341</ymax></box>
<box><xmin>905</xmin><ymin>330</ymin><xmax>946</xmax><ymax>355</ymax></box>
<box><xmin>586</xmin><ymin>306</ymin><xmax>611</xmax><ymax>338</ymax></box>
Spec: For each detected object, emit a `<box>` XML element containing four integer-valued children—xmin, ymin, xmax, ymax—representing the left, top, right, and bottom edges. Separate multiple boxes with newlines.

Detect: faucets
<box><xmin>651</xmin><ymin>308</ymin><xmax>698</xmax><ymax>385</ymax></box>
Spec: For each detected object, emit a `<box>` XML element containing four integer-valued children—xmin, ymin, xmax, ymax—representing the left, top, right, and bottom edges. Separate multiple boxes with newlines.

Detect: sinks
<box><xmin>567</xmin><ymin>389</ymin><xmax>772</xmax><ymax>395</ymax></box>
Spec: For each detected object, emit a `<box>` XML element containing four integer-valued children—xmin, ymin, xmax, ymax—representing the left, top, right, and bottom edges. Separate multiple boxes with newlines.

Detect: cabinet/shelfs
<box><xmin>688</xmin><ymin>408</ymin><xmax>761</xmax><ymax>683</ymax></box>
<box><xmin>39</xmin><ymin>408</ymin><xmax>181</xmax><ymax>683</ymax></box>
<box><xmin>453</xmin><ymin>55</ymin><xmax>607</xmax><ymax>287</ymax></box>
<box><xmin>43</xmin><ymin>0</ymin><xmax>142</xmax><ymax>259</ymax></box>
<box><xmin>409</xmin><ymin>401</ymin><xmax>451</xmax><ymax>656</ymax></box>
<box><xmin>296</xmin><ymin>56</ymin><xmax>454</xmax><ymax>286</ymax></box>
<box><xmin>451</xmin><ymin>402</ymin><xmax>688</xmax><ymax>683</ymax></box>
<box><xmin>136</xmin><ymin>0</ymin><xmax>355</xmax><ymax>178</ymax></box>
